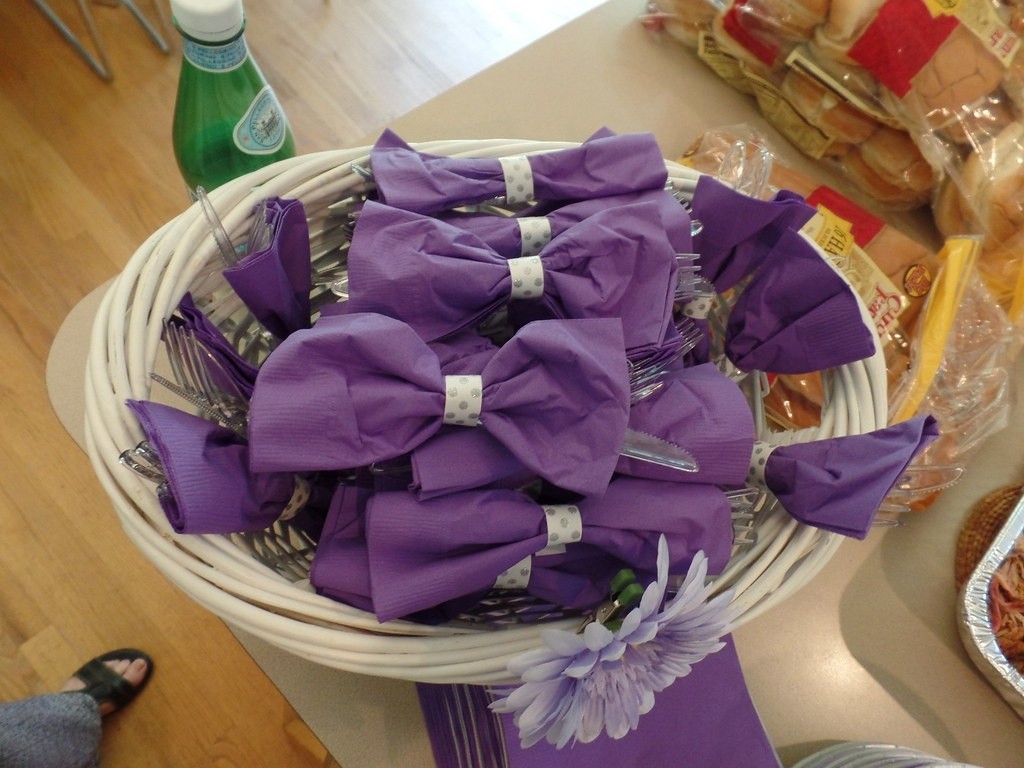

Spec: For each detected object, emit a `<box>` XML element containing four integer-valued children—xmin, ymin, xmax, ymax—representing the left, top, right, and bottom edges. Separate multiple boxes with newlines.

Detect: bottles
<box><xmin>171</xmin><ymin>1</ymin><xmax>298</xmax><ymax>253</ymax></box>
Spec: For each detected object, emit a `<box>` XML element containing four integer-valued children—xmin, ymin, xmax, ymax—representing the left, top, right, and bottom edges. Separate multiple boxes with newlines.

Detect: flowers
<box><xmin>483</xmin><ymin>534</ymin><xmax>748</xmax><ymax>750</ymax></box>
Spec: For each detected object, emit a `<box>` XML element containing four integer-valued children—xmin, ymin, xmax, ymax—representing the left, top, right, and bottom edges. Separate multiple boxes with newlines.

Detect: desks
<box><xmin>48</xmin><ymin>1</ymin><xmax>1024</xmax><ymax>768</ymax></box>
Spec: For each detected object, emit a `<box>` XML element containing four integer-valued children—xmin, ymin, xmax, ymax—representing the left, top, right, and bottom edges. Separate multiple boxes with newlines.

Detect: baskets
<box><xmin>792</xmin><ymin>743</ymin><xmax>978</xmax><ymax>768</ymax></box>
<box><xmin>87</xmin><ymin>139</ymin><xmax>888</xmax><ymax>683</ymax></box>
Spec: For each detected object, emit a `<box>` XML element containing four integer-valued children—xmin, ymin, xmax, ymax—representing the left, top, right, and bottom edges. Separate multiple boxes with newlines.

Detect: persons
<box><xmin>1</xmin><ymin>648</ymin><xmax>154</xmax><ymax>768</ymax></box>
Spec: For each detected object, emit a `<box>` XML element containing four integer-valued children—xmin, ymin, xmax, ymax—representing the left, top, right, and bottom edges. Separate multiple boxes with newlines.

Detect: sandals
<box><xmin>61</xmin><ymin>648</ymin><xmax>154</xmax><ymax>718</ymax></box>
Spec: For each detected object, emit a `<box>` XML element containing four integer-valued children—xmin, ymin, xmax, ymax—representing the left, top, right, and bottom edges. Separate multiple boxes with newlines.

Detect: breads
<box><xmin>646</xmin><ymin>0</ymin><xmax>1024</xmax><ymax>512</ymax></box>
